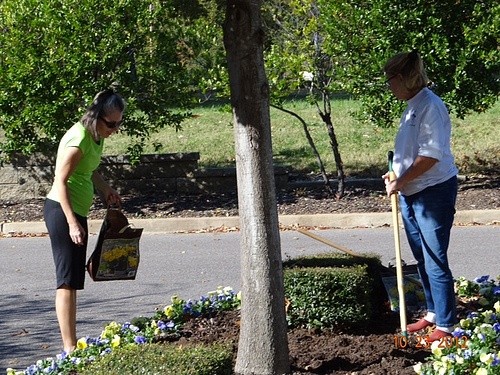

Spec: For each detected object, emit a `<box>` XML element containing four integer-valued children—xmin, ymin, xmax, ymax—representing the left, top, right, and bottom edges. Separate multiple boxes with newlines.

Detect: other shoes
<box><xmin>428</xmin><ymin>329</ymin><xmax>451</xmax><ymax>342</ymax></box>
<box><xmin>406</xmin><ymin>318</ymin><xmax>436</xmax><ymax>332</ymax></box>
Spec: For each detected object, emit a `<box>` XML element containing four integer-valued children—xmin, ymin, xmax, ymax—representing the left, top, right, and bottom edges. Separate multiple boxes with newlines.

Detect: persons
<box><xmin>382</xmin><ymin>51</ymin><xmax>464</xmax><ymax>346</ymax></box>
<box><xmin>41</xmin><ymin>87</ymin><xmax>131</xmax><ymax>357</ymax></box>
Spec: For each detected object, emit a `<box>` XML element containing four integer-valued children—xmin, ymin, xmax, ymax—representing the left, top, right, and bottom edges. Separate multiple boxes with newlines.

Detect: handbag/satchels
<box><xmin>85</xmin><ymin>195</ymin><xmax>143</xmax><ymax>282</ymax></box>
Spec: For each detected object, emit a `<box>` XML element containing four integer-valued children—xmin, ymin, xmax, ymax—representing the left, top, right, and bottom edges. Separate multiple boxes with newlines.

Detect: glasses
<box><xmin>98</xmin><ymin>115</ymin><xmax>124</xmax><ymax>129</ymax></box>
<box><xmin>384</xmin><ymin>74</ymin><xmax>397</xmax><ymax>88</ymax></box>
